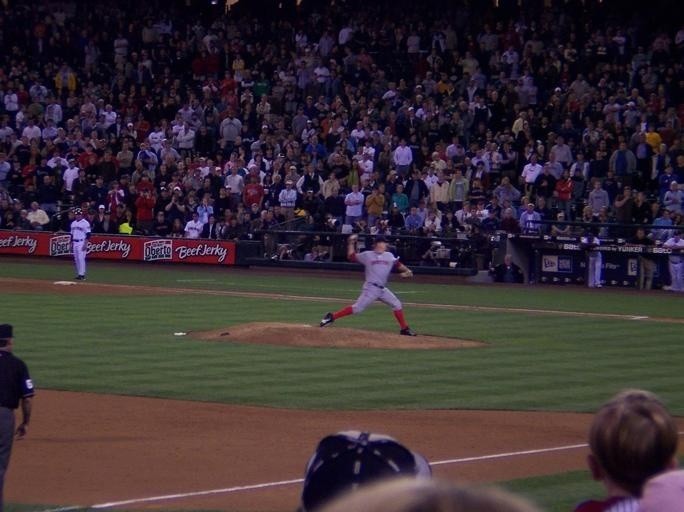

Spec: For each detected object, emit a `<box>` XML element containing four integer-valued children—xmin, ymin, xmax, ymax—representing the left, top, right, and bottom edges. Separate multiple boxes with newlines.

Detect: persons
<box><xmin>0</xmin><ymin>0</ymin><xmax>683</xmax><ymax>293</ymax></box>
<box><xmin>320</xmin><ymin>234</ymin><xmax>419</xmax><ymax>337</ymax></box>
<box><xmin>0</xmin><ymin>323</ymin><xmax>34</xmax><ymax>508</ymax></box>
<box><xmin>568</xmin><ymin>387</ymin><xmax>678</xmax><ymax>512</ymax></box>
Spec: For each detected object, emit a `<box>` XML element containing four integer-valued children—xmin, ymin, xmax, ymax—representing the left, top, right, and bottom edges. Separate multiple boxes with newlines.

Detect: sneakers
<box><xmin>400</xmin><ymin>328</ymin><xmax>415</xmax><ymax>336</ymax></box>
<box><xmin>320</xmin><ymin>313</ymin><xmax>332</xmax><ymax>326</ymax></box>
